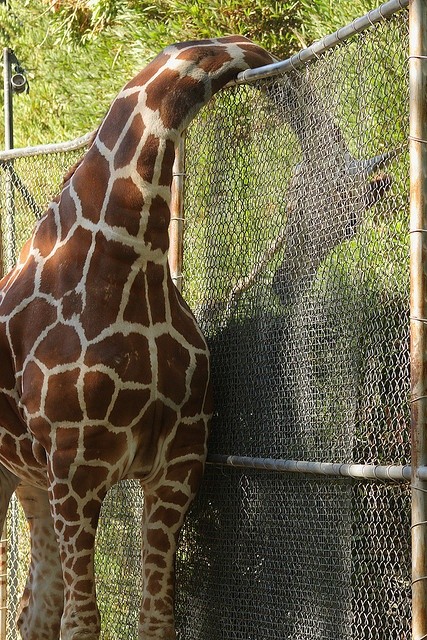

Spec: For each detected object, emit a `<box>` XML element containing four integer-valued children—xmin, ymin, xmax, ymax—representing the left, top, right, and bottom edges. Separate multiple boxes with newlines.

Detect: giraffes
<box><xmin>0</xmin><ymin>36</ymin><xmax>394</xmax><ymax>640</ymax></box>
